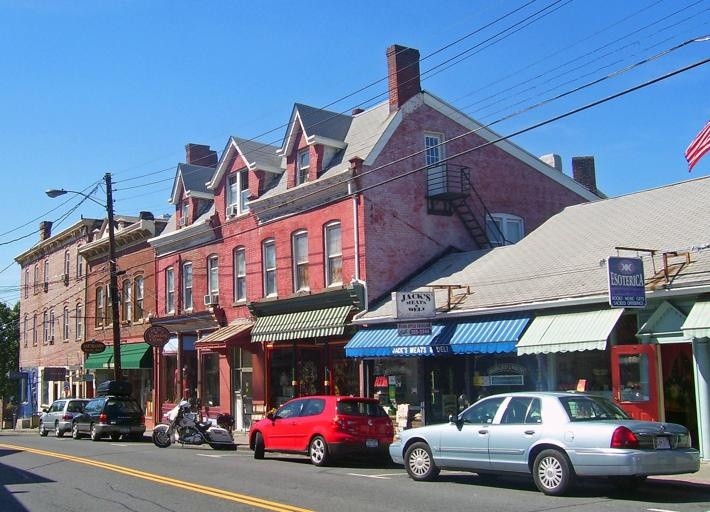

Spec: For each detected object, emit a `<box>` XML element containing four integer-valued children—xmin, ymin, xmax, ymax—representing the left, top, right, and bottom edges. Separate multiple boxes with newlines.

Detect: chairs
<box><xmin>306</xmin><ymin>405</ymin><xmax>319</xmax><ymax>415</ymax></box>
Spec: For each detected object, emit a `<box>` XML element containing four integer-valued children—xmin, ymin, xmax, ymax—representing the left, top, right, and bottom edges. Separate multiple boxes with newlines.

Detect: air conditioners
<box><xmin>48</xmin><ymin>335</ymin><xmax>54</xmax><ymax>341</ymax></box>
<box><xmin>61</xmin><ymin>274</ymin><xmax>69</xmax><ymax>281</ymax></box>
<box><xmin>203</xmin><ymin>294</ymin><xmax>219</xmax><ymax>305</ymax></box>
<box><xmin>225</xmin><ymin>206</ymin><xmax>236</xmax><ymax>216</ymax></box>
<box><xmin>178</xmin><ymin>216</ymin><xmax>188</xmax><ymax>227</ymax></box>
<box><xmin>42</xmin><ymin>282</ymin><xmax>47</xmax><ymax>289</ymax></box>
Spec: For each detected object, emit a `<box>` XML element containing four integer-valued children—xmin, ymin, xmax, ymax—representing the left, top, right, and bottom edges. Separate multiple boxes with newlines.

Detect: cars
<box><xmin>70</xmin><ymin>397</ymin><xmax>146</xmax><ymax>441</ymax></box>
<box><xmin>38</xmin><ymin>399</ymin><xmax>93</xmax><ymax>438</ymax></box>
<box><xmin>248</xmin><ymin>396</ymin><xmax>394</xmax><ymax>466</ymax></box>
<box><xmin>389</xmin><ymin>390</ymin><xmax>701</xmax><ymax>497</ymax></box>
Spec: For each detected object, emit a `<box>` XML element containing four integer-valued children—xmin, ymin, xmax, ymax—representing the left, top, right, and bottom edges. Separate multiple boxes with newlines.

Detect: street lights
<box><xmin>44</xmin><ymin>172</ymin><xmax>123</xmax><ymax>397</ymax></box>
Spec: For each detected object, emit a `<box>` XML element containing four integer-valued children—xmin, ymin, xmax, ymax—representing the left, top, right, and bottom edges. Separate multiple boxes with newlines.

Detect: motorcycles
<box><xmin>152</xmin><ymin>398</ymin><xmax>238</xmax><ymax>450</ymax></box>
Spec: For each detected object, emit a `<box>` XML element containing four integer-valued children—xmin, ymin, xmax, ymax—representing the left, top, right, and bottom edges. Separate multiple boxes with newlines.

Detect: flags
<box><xmin>683</xmin><ymin>121</ymin><xmax>710</xmax><ymax>174</ymax></box>
